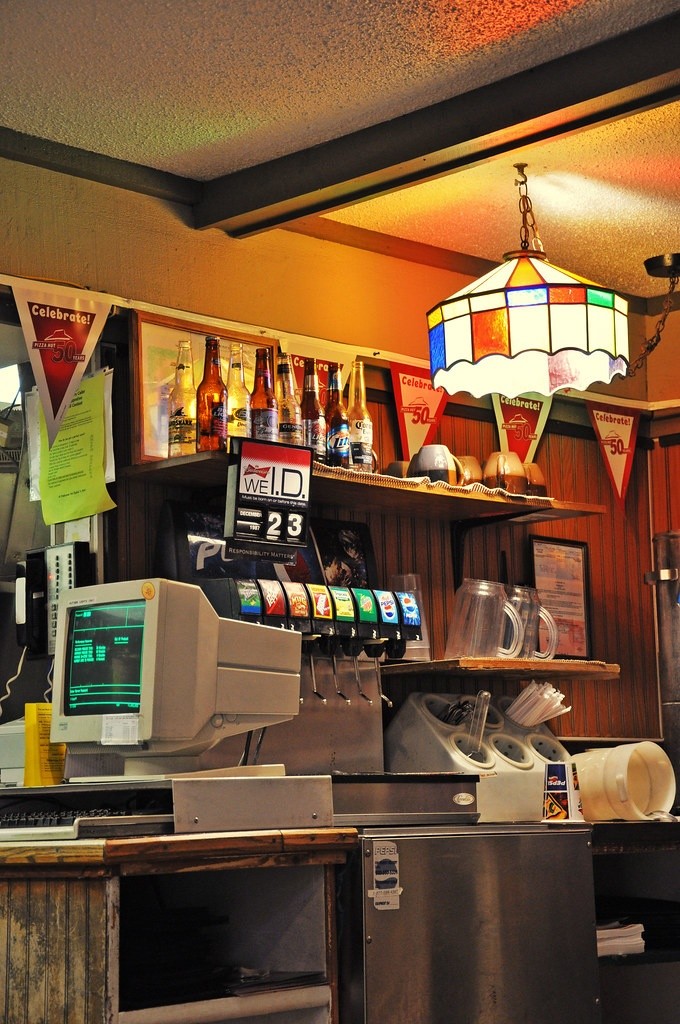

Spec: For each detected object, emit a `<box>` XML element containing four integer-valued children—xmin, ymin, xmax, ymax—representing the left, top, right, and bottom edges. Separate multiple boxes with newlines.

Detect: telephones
<box><xmin>14</xmin><ymin>541</ymin><xmax>91</xmax><ymax>660</ymax></box>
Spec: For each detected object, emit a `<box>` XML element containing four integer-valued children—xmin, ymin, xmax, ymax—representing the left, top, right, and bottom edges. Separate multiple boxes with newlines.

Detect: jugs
<box><xmin>503</xmin><ymin>585</ymin><xmax>558</xmax><ymax>661</ymax></box>
<box><xmin>444</xmin><ymin>578</ymin><xmax>524</xmax><ymax>659</ymax></box>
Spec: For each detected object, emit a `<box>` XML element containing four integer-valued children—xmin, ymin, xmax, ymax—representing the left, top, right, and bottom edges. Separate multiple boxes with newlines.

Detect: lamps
<box><xmin>424</xmin><ymin>161</ymin><xmax>630</xmax><ymax>401</ymax></box>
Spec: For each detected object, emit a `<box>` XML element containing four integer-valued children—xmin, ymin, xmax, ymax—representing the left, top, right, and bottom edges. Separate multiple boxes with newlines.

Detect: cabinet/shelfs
<box><xmin>132</xmin><ymin>310</ymin><xmax>622</xmax><ymax>686</ymax></box>
<box><xmin>0</xmin><ymin>827</ymin><xmax>362</xmax><ymax>1024</ymax></box>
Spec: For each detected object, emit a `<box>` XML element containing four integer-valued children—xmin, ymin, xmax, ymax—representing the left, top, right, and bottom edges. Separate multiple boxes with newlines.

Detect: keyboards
<box><xmin>0</xmin><ymin>808</ymin><xmax>132</xmax><ymax>826</ymax></box>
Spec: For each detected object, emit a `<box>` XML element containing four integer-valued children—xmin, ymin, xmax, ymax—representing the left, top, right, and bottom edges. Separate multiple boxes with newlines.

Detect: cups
<box><xmin>455</xmin><ymin>455</ymin><xmax>483</xmax><ymax>487</ymax></box>
<box><xmin>406</xmin><ymin>453</ymin><xmax>420</xmax><ymax>478</ymax></box>
<box><xmin>382</xmin><ymin>461</ymin><xmax>411</xmax><ymax>478</ymax></box>
<box><xmin>523</xmin><ymin>463</ymin><xmax>547</xmax><ymax>497</ymax></box>
<box><xmin>416</xmin><ymin>444</ymin><xmax>464</xmax><ymax>486</ymax></box>
<box><xmin>372</xmin><ymin>449</ymin><xmax>378</xmax><ymax>473</ymax></box>
<box><xmin>483</xmin><ymin>452</ymin><xmax>528</xmax><ymax>494</ymax></box>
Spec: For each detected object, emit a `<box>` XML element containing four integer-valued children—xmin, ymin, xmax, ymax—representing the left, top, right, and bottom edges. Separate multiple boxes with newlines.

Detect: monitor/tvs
<box><xmin>49</xmin><ymin>577</ymin><xmax>302</xmax><ymax>782</ymax></box>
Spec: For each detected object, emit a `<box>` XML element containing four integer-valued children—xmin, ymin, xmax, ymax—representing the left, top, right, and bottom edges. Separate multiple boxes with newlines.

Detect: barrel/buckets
<box><xmin>572</xmin><ymin>741</ymin><xmax>676</xmax><ymax>820</ymax></box>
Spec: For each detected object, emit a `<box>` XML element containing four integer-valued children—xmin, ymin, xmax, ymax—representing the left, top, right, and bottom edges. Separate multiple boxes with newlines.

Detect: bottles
<box><xmin>275</xmin><ymin>353</ymin><xmax>301</xmax><ymax>446</ymax></box>
<box><xmin>196</xmin><ymin>336</ymin><xmax>228</xmax><ymax>454</ymax></box>
<box><xmin>346</xmin><ymin>362</ymin><xmax>373</xmax><ymax>472</ymax></box>
<box><xmin>299</xmin><ymin>358</ymin><xmax>326</xmax><ymax>463</ymax></box>
<box><xmin>250</xmin><ymin>348</ymin><xmax>279</xmax><ymax>442</ymax></box>
<box><xmin>225</xmin><ymin>343</ymin><xmax>251</xmax><ymax>454</ymax></box>
<box><xmin>167</xmin><ymin>340</ymin><xmax>196</xmax><ymax>458</ymax></box>
<box><xmin>324</xmin><ymin>362</ymin><xmax>349</xmax><ymax>469</ymax></box>
